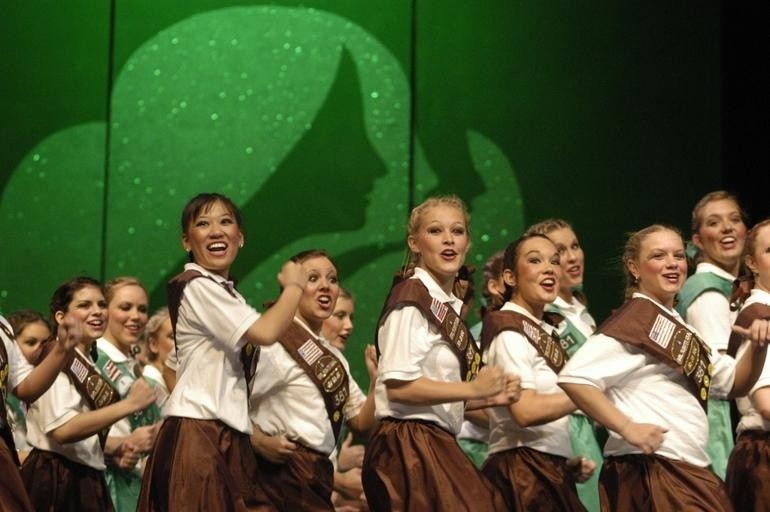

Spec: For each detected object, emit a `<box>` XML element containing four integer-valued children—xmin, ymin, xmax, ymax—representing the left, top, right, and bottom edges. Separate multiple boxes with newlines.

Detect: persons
<box><xmin>0</xmin><ymin>191</ymin><xmax>770</xmax><ymax>512</ymax></box>
